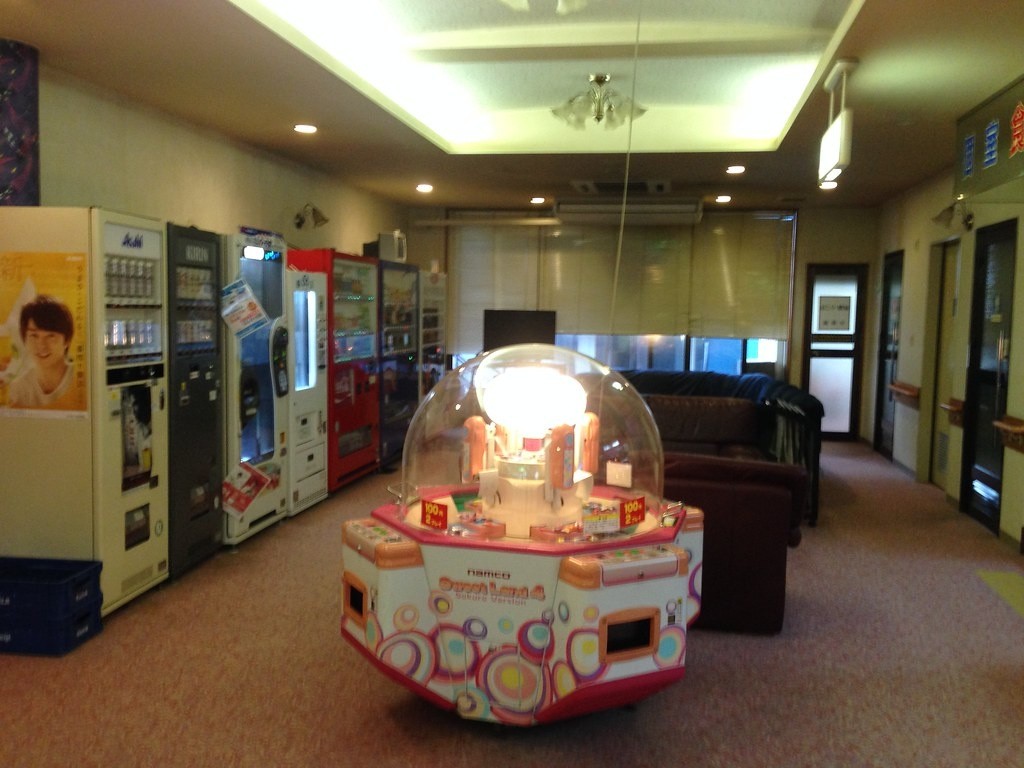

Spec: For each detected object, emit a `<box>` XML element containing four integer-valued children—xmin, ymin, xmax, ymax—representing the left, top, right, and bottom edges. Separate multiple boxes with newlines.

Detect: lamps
<box><xmin>549</xmin><ymin>72</ymin><xmax>648</xmax><ymax>131</ymax></box>
<box><xmin>930</xmin><ymin>200</ymin><xmax>974</xmax><ymax>231</ymax></box>
<box><xmin>295</xmin><ymin>201</ymin><xmax>329</xmax><ymax>230</ymax></box>
<box><xmin>817</xmin><ymin>57</ymin><xmax>860</xmax><ymax>190</ymax></box>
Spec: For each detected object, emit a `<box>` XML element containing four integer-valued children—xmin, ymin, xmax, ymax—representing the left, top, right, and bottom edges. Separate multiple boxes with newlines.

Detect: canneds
<box><xmin>100</xmin><ymin>258</ymin><xmax>156</xmax><ymax>347</ymax></box>
<box><xmin>173</xmin><ymin>266</ymin><xmax>213</xmax><ymax>343</ymax></box>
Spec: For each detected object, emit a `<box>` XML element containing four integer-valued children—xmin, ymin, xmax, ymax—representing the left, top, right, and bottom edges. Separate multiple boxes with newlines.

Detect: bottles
<box><xmin>104</xmin><ymin>257</ymin><xmax>155</xmax><ymax>297</ymax></box>
<box><xmin>105</xmin><ymin>321</ymin><xmax>153</xmax><ymax>345</ymax></box>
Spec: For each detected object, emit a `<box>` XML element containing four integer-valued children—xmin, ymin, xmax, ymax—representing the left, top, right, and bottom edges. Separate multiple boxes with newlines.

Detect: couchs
<box><xmin>576</xmin><ymin>370</ymin><xmax>824</xmax><ymax>526</ymax></box>
<box><xmin>614</xmin><ymin>451</ymin><xmax>807</xmax><ymax>637</ymax></box>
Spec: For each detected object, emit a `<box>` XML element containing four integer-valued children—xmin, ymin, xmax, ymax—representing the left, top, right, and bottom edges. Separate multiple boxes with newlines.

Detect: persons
<box><xmin>9</xmin><ymin>295</ymin><xmax>86</xmax><ymax>411</ymax></box>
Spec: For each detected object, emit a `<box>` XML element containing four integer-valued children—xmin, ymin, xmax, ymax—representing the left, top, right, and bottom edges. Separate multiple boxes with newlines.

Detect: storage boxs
<box><xmin>0</xmin><ymin>557</ymin><xmax>104</xmax><ymax>657</ymax></box>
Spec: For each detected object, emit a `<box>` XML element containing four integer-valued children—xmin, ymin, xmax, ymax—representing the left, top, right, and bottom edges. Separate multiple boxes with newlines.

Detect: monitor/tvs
<box><xmin>363</xmin><ymin>232</ymin><xmax>406</xmax><ymax>264</ymax></box>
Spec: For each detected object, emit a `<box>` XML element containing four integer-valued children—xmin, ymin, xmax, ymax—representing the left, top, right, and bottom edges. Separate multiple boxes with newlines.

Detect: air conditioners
<box><xmin>553</xmin><ymin>197</ymin><xmax>703</xmax><ymax>228</ymax></box>
